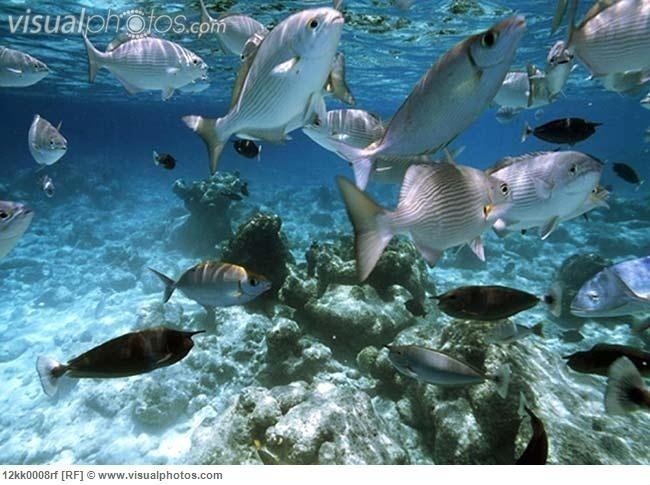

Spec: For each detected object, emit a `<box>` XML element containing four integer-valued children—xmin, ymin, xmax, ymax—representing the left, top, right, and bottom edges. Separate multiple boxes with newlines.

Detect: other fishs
<box><xmin>1</xmin><ymin>0</ymin><xmax>650</xmax><ymax>465</ymax></box>
